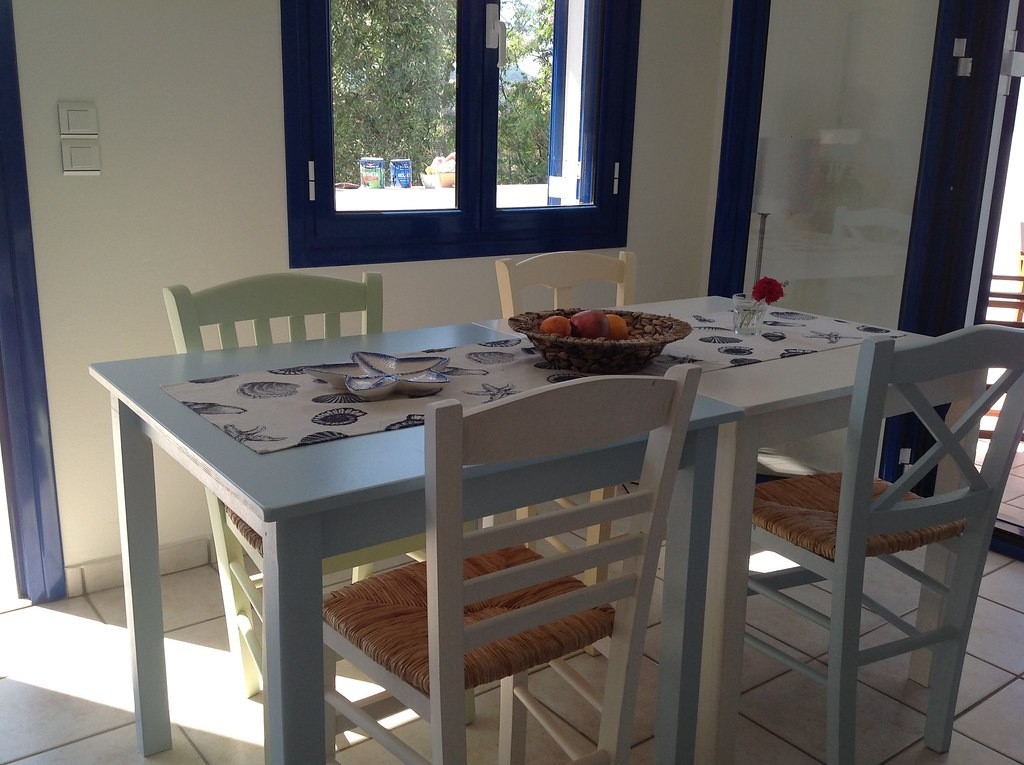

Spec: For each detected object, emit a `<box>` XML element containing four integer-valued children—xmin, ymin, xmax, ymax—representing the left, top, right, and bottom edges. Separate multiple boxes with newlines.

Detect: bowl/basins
<box><xmin>425</xmin><ymin>168</ymin><xmax>455</xmax><ymax>187</ymax></box>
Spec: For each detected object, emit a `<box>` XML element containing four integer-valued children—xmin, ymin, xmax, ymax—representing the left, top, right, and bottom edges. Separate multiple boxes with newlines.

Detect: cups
<box><xmin>733</xmin><ymin>293</ymin><xmax>767</xmax><ymax>335</ymax></box>
<box><xmin>420</xmin><ymin>172</ymin><xmax>439</xmax><ymax>189</ymax></box>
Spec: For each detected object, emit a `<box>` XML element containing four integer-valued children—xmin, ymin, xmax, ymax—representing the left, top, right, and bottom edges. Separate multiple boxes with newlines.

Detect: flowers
<box><xmin>752</xmin><ymin>276</ymin><xmax>791</xmax><ymax>303</ymax></box>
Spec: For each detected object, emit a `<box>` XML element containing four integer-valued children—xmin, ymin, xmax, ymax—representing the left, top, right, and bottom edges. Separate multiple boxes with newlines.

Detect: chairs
<box><xmin>497</xmin><ymin>250</ymin><xmax>638</xmax><ymax>655</ymax></box>
<box><xmin>160</xmin><ymin>273</ymin><xmax>483</xmax><ymax>699</ymax></box>
<box><xmin>746</xmin><ymin>323</ymin><xmax>1023</xmax><ymax>765</ymax></box>
<box><xmin>320</xmin><ymin>363</ymin><xmax>703</xmax><ymax>765</ymax></box>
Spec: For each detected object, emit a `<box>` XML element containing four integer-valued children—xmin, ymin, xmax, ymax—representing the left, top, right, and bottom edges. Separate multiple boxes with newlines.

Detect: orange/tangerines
<box><xmin>606</xmin><ymin>315</ymin><xmax>628</xmax><ymax>339</ymax></box>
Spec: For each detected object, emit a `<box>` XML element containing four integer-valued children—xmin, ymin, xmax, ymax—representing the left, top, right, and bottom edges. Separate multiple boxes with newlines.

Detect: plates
<box><xmin>303</xmin><ymin>351</ymin><xmax>450</xmax><ymax>401</ymax></box>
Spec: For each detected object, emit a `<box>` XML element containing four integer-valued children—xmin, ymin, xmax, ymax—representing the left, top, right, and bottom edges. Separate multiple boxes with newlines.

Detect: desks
<box><xmin>335</xmin><ymin>184</ymin><xmax>548</xmax><ymax>211</ymax></box>
<box><xmin>87</xmin><ymin>295</ymin><xmax>988</xmax><ymax>765</ymax></box>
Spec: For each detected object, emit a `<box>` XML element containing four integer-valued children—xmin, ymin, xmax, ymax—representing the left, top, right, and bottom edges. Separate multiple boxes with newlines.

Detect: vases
<box><xmin>732</xmin><ymin>293</ymin><xmax>768</xmax><ymax>335</ymax></box>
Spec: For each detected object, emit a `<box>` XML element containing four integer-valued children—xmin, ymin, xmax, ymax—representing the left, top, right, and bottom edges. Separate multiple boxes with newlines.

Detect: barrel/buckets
<box><xmin>390</xmin><ymin>159</ymin><xmax>412</xmax><ymax>188</ymax></box>
<box><xmin>359</xmin><ymin>157</ymin><xmax>385</xmax><ymax>189</ymax></box>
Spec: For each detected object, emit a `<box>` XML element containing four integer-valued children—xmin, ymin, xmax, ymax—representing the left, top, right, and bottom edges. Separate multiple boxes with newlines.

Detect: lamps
<box><xmin>750</xmin><ymin>138</ymin><xmax>781</xmax><ymax>285</ymax></box>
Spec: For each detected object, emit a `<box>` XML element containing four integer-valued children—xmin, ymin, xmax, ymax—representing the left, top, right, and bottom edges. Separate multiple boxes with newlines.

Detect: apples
<box><xmin>570</xmin><ymin>310</ymin><xmax>609</xmax><ymax>339</ymax></box>
<box><xmin>540</xmin><ymin>316</ymin><xmax>571</xmax><ymax>336</ymax></box>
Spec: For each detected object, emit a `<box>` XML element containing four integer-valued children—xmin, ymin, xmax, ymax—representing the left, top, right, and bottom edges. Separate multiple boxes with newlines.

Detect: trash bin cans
<box><xmin>359</xmin><ymin>156</ymin><xmax>386</xmax><ymax>188</ymax></box>
<box><xmin>390</xmin><ymin>159</ymin><xmax>412</xmax><ymax>188</ymax></box>
